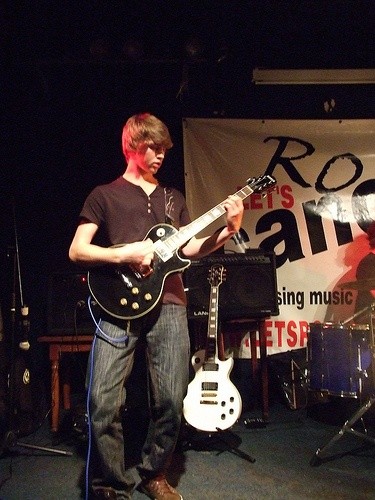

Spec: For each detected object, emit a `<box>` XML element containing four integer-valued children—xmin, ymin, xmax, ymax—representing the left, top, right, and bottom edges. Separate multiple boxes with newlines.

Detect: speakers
<box><xmin>46</xmin><ymin>271</ymin><xmax>98</xmax><ymax>336</ymax></box>
<box><xmin>182</xmin><ymin>250</ymin><xmax>280</xmax><ymax>320</ymax></box>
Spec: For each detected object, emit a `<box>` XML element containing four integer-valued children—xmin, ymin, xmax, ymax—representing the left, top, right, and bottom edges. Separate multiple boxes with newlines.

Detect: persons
<box><xmin>68</xmin><ymin>112</ymin><xmax>244</xmax><ymax>500</ymax></box>
<box><xmin>353</xmin><ymin>221</ymin><xmax>375</xmax><ymax>324</ymax></box>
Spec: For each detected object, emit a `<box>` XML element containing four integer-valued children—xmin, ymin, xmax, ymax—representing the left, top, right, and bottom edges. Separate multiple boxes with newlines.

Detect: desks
<box><xmin>218</xmin><ymin>316</ymin><xmax>270</xmax><ymax>418</ymax></box>
<box><xmin>38</xmin><ymin>334</ymin><xmax>152</xmax><ymax>432</ymax></box>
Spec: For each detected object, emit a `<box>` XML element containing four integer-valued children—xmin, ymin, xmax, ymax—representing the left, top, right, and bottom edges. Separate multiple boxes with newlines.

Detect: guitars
<box><xmin>86</xmin><ymin>174</ymin><xmax>277</xmax><ymax>321</ymax></box>
<box><xmin>182</xmin><ymin>264</ymin><xmax>243</xmax><ymax>432</ymax></box>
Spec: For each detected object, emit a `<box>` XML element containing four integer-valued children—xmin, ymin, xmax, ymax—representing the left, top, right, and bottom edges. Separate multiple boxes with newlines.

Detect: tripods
<box><xmin>0</xmin><ymin>247</ymin><xmax>74</xmax><ymax>459</ymax></box>
<box><xmin>310</xmin><ymin>312</ymin><xmax>375</xmax><ymax>467</ymax></box>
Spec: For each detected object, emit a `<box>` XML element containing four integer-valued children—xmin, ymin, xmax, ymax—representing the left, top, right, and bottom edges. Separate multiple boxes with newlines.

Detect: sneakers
<box><xmin>93</xmin><ymin>488</ymin><xmax>118</xmax><ymax>500</ymax></box>
<box><xmin>141</xmin><ymin>471</ymin><xmax>183</xmax><ymax>500</ymax></box>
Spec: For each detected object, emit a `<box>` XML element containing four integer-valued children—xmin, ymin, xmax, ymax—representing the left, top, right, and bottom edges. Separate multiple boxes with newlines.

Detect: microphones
<box><xmin>7</xmin><ymin>192</ymin><xmax>17</xmax><ymax>217</ymax></box>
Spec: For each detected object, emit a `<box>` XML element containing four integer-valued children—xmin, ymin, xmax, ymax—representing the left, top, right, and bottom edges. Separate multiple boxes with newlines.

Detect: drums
<box><xmin>307</xmin><ymin>322</ymin><xmax>371</xmax><ymax>400</ymax></box>
